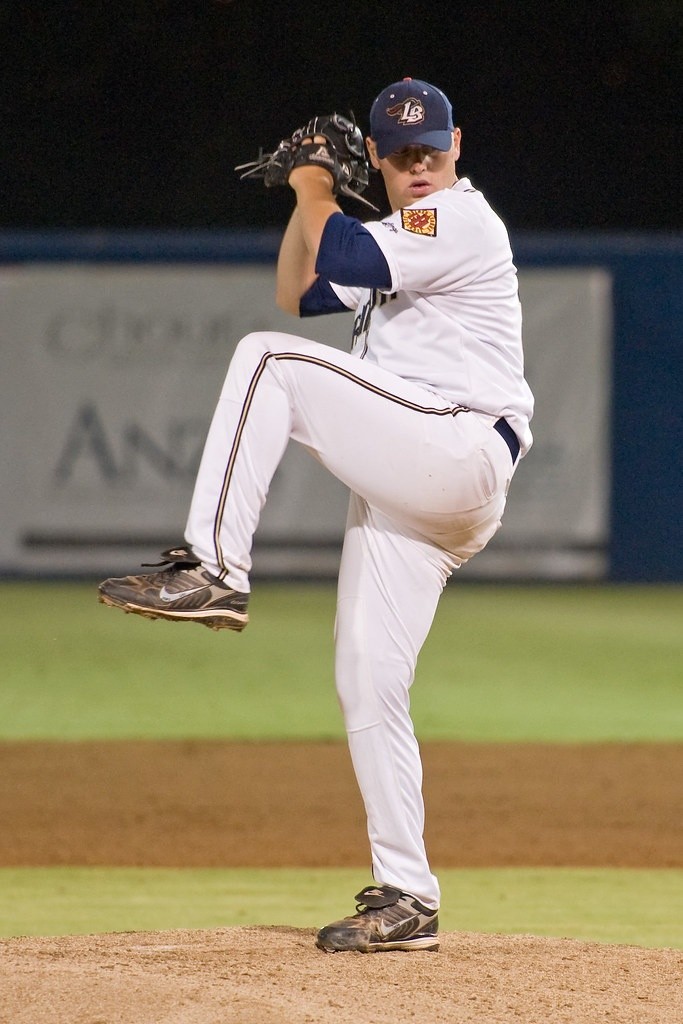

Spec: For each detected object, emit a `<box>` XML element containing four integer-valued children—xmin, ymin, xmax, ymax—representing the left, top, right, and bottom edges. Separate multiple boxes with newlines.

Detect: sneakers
<box><xmin>316</xmin><ymin>885</ymin><xmax>441</xmax><ymax>954</ymax></box>
<box><xmin>98</xmin><ymin>546</ymin><xmax>249</xmax><ymax>633</ymax></box>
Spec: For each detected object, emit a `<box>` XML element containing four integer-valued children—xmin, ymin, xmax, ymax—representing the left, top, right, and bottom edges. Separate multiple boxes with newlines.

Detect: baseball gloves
<box><xmin>264</xmin><ymin>114</ymin><xmax>369</xmax><ymax>196</ymax></box>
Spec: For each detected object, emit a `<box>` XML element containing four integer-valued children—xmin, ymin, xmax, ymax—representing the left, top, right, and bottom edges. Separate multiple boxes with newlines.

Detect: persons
<box><xmin>96</xmin><ymin>78</ymin><xmax>534</xmax><ymax>954</ymax></box>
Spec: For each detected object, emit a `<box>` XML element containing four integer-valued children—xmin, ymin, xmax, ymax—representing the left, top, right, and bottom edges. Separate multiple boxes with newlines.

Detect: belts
<box><xmin>495</xmin><ymin>416</ymin><xmax>521</xmax><ymax>466</ymax></box>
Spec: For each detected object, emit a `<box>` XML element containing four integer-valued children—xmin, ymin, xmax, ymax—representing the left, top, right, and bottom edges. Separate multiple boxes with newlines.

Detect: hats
<box><xmin>370</xmin><ymin>77</ymin><xmax>455</xmax><ymax>158</ymax></box>
